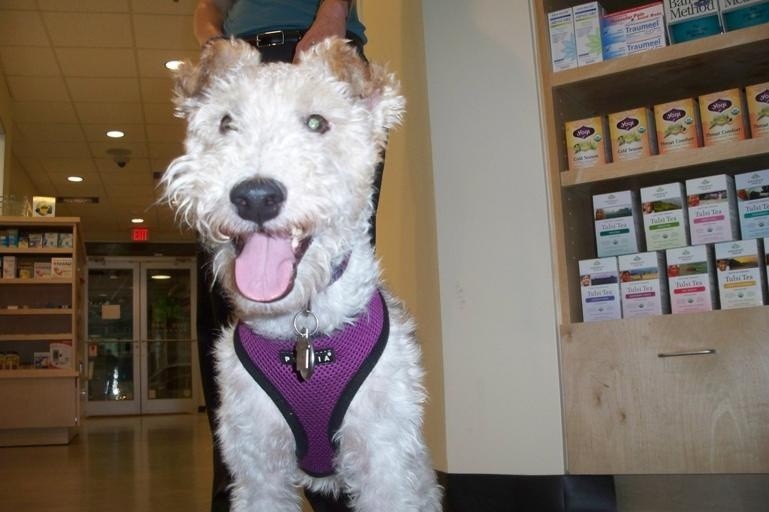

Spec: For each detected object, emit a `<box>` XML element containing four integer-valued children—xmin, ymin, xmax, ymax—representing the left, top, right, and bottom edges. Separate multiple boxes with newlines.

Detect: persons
<box><xmin>688</xmin><ymin>195</ymin><xmax>700</xmax><ymax>207</ymax></box>
<box><xmin>717</xmin><ymin>259</ymin><xmax>730</xmax><ymax>273</ymax></box>
<box><xmin>595</xmin><ymin>208</ymin><xmax>604</xmax><ymax>220</ymax></box>
<box><xmin>620</xmin><ymin>271</ymin><xmax>631</xmax><ymax>282</ymax></box>
<box><xmin>643</xmin><ymin>202</ymin><xmax>655</xmax><ymax>215</ymax></box>
<box><xmin>737</xmin><ymin>190</ymin><xmax>749</xmax><ymax>201</ymax></box>
<box><xmin>580</xmin><ymin>275</ymin><xmax>590</xmax><ymax>287</ymax></box>
<box><xmin>191</xmin><ymin>0</ymin><xmax>384</xmax><ymax>512</ymax></box>
<box><xmin>669</xmin><ymin>265</ymin><xmax>679</xmax><ymax>277</ymax></box>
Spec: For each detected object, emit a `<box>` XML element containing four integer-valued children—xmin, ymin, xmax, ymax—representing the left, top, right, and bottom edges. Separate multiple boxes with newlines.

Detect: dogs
<box><xmin>155</xmin><ymin>36</ymin><xmax>448</xmax><ymax>511</ymax></box>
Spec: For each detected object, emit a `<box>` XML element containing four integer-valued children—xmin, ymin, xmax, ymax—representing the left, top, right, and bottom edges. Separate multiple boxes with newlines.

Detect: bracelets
<box><xmin>199</xmin><ymin>34</ymin><xmax>225</xmax><ymax>52</ymax></box>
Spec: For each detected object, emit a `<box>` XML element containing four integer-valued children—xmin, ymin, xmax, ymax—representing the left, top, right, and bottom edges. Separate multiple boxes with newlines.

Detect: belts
<box><xmin>245</xmin><ymin>29</ymin><xmax>302</xmax><ymax>48</ymax></box>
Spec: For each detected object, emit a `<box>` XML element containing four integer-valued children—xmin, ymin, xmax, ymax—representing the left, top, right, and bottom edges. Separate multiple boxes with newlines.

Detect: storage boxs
<box><xmin>617</xmin><ymin>251</ymin><xmax>670</xmax><ymax>318</ymax></box>
<box><xmin>665</xmin><ymin>245</ymin><xmax>717</xmax><ymax>314</ymax></box>
<box><xmin>654</xmin><ymin>97</ymin><xmax>704</xmax><ymax>154</ymax></box>
<box><xmin>640</xmin><ymin>182</ymin><xmax>691</xmax><ymax>252</ymax></box>
<box><xmin>601</xmin><ymin>15</ymin><xmax>664</xmax><ymax>45</ymax></box>
<box><xmin>714</xmin><ymin>238</ymin><xmax>765</xmax><ymax>310</ymax></box>
<box><xmin>564</xmin><ymin>115</ymin><xmax>612</xmax><ymax>170</ymax></box>
<box><xmin>578</xmin><ymin>256</ymin><xmax>623</xmax><ymax>322</ymax></box>
<box><xmin>592</xmin><ymin>190</ymin><xmax>646</xmax><ymax>259</ymax></box>
<box><xmin>698</xmin><ymin>88</ymin><xmax>749</xmax><ymax>147</ymax></box>
<box><xmin>602</xmin><ymin>32</ymin><xmax>666</xmax><ymax>60</ymax></box>
<box><xmin>602</xmin><ymin>2</ymin><xmax>665</xmax><ymax>26</ymax></box>
<box><xmin>745</xmin><ymin>82</ymin><xmax>768</xmax><ymax>138</ymax></box>
<box><xmin>734</xmin><ymin>169</ymin><xmax>768</xmax><ymax>240</ymax></box>
<box><xmin>664</xmin><ymin>0</ymin><xmax>723</xmax><ymax>45</ymax></box>
<box><xmin>686</xmin><ymin>174</ymin><xmax>740</xmax><ymax>246</ymax></box>
<box><xmin>548</xmin><ymin>7</ymin><xmax>578</xmax><ymax>74</ymax></box>
<box><xmin>609</xmin><ymin>107</ymin><xmax>658</xmax><ymax>163</ymax></box>
<box><xmin>718</xmin><ymin>0</ymin><xmax>768</xmax><ymax>32</ymax></box>
<box><xmin>763</xmin><ymin>237</ymin><xmax>768</xmax><ymax>280</ymax></box>
<box><xmin>572</xmin><ymin>1</ymin><xmax>604</xmax><ymax>67</ymax></box>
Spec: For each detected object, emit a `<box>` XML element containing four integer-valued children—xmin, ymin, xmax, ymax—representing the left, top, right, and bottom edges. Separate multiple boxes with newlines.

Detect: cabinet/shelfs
<box><xmin>527</xmin><ymin>0</ymin><xmax>769</xmax><ymax>511</ymax></box>
<box><xmin>1</xmin><ymin>218</ymin><xmax>89</xmax><ymax>448</ymax></box>
